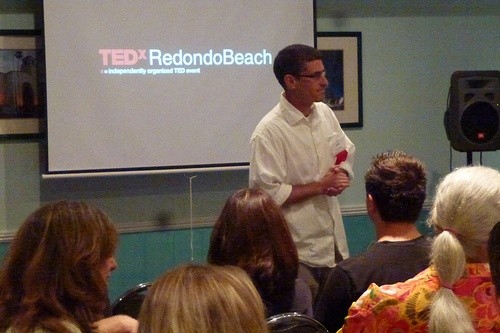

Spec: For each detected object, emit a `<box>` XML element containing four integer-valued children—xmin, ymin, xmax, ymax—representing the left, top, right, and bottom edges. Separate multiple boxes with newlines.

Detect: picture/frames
<box><xmin>315</xmin><ymin>30</ymin><xmax>364</xmax><ymax>130</ymax></box>
<box><xmin>0</xmin><ymin>29</ymin><xmax>45</xmax><ymax>140</ymax></box>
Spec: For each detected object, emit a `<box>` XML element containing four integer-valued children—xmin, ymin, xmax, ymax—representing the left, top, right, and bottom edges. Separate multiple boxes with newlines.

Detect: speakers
<box><xmin>443</xmin><ymin>70</ymin><xmax>500</xmax><ymax>152</ymax></box>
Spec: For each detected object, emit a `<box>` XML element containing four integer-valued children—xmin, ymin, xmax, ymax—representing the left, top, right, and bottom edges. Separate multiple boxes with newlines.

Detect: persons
<box><xmin>337</xmin><ymin>164</ymin><xmax>500</xmax><ymax>333</ymax></box>
<box><xmin>487</xmin><ymin>222</ymin><xmax>500</xmax><ymax>300</ymax></box>
<box><xmin>138</xmin><ymin>262</ymin><xmax>269</xmax><ymax>333</ymax></box>
<box><xmin>0</xmin><ymin>200</ymin><xmax>138</xmax><ymax>333</ymax></box>
<box><xmin>315</xmin><ymin>149</ymin><xmax>432</xmax><ymax>333</ymax></box>
<box><xmin>248</xmin><ymin>43</ymin><xmax>356</xmax><ymax>309</ymax></box>
<box><xmin>207</xmin><ymin>188</ymin><xmax>319</xmax><ymax>321</ymax></box>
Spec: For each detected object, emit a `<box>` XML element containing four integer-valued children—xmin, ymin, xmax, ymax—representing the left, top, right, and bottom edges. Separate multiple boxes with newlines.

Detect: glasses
<box><xmin>295</xmin><ymin>69</ymin><xmax>328</xmax><ymax>80</ymax></box>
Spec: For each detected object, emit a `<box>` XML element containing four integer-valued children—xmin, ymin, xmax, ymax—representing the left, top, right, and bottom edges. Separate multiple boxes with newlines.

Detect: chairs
<box><xmin>110</xmin><ymin>280</ymin><xmax>153</xmax><ymax>321</ymax></box>
<box><xmin>265</xmin><ymin>312</ymin><xmax>329</xmax><ymax>333</ymax></box>
